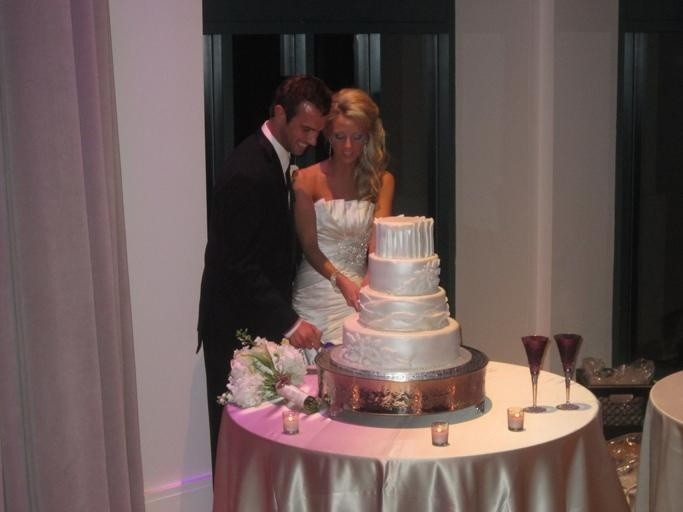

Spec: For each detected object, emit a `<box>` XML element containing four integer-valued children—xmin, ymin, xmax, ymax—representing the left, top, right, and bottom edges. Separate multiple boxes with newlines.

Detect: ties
<box><xmin>281</xmin><ymin>160</ymin><xmax>299</xmax><ymax>213</ymax></box>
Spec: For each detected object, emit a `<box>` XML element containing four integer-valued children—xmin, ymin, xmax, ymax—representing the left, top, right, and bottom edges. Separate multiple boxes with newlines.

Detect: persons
<box><xmin>290</xmin><ymin>86</ymin><xmax>396</xmax><ymax>346</ymax></box>
<box><xmin>192</xmin><ymin>73</ymin><xmax>334</xmax><ymax>485</ymax></box>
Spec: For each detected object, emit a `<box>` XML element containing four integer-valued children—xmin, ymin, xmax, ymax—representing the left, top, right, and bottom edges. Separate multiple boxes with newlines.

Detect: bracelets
<box><xmin>330</xmin><ymin>269</ymin><xmax>347</xmax><ymax>295</ymax></box>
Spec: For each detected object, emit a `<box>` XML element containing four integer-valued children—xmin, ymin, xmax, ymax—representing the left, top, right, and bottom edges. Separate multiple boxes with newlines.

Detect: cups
<box><xmin>507</xmin><ymin>407</ymin><xmax>524</xmax><ymax>431</ymax></box>
<box><xmin>280</xmin><ymin>409</ymin><xmax>301</xmax><ymax>435</ymax></box>
<box><xmin>582</xmin><ymin>358</ymin><xmax>655</xmax><ymax>401</ymax></box>
<box><xmin>430</xmin><ymin>421</ymin><xmax>448</xmax><ymax>447</ymax></box>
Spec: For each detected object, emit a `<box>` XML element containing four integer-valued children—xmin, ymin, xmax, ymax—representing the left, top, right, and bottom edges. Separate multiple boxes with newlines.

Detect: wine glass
<box><xmin>552</xmin><ymin>333</ymin><xmax>583</xmax><ymax>410</ymax></box>
<box><xmin>520</xmin><ymin>336</ymin><xmax>548</xmax><ymax>414</ymax></box>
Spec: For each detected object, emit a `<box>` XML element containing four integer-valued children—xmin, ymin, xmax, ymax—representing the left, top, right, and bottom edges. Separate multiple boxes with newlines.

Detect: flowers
<box><xmin>215</xmin><ymin>327</ymin><xmax>320</xmax><ymax>415</ymax></box>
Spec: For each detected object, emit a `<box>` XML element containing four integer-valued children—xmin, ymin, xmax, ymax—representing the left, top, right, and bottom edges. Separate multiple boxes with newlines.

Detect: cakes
<box><xmin>341</xmin><ymin>216</ymin><xmax>461</xmax><ymax>371</ymax></box>
